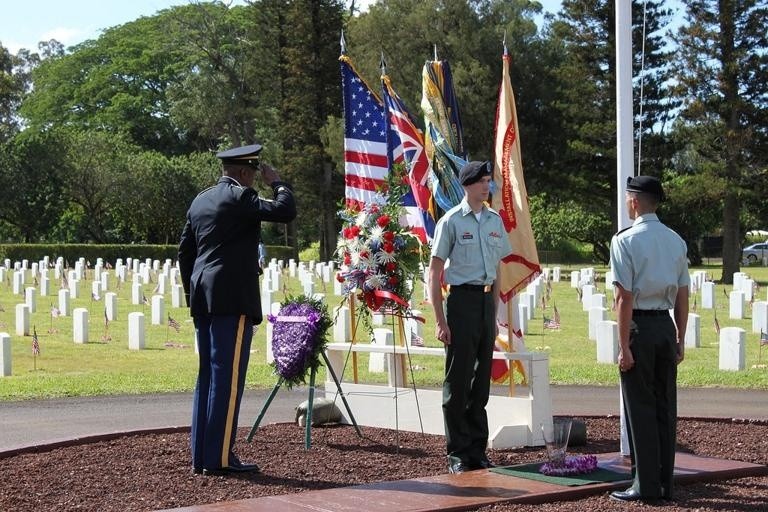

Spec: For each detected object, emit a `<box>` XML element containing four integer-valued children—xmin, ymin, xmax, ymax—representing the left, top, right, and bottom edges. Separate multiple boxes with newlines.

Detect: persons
<box><xmin>177</xmin><ymin>143</ymin><xmax>297</xmax><ymax>477</ymax></box>
<box><xmin>610</xmin><ymin>175</ymin><xmax>692</xmax><ymax>503</ymax></box>
<box><xmin>427</xmin><ymin>159</ymin><xmax>514</xmax><ymax>473</ymax></box>
<box><xmin>258</xmin><ymin>239</ymin><xmax>266</xmax><ymax>274</ymax></box>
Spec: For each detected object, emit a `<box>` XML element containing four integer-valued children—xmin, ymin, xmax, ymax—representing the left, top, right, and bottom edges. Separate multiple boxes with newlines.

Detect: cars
<box><xmin>742</xmin><ymin>243</ymin><xmax>766</xmax><ymax>264</ymax></box>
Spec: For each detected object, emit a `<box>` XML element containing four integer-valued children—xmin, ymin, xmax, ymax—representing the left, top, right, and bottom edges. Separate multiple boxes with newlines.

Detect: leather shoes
<box><xmin>202</xmin><ymin>457</ymin><xmax>259</xmax><ymax>476</ymax></box>
<box><xmin>193</xmin><ymin>467</ymin><xmax>203</xmax><ymax>474</ymax></box>
<box><xmin>609</xmin><ymin>485</ymin><xmax>658</xmax><ymax>505</ymax></box>
<box><xmin>474</xmin><ymin>461</ymin><xmax>496</xmax><ymax>470</ymax></box>
<box><xmin>449</xmin><ymin>463</ymin><xmax>474</xmax><ymax>474</ymax></box>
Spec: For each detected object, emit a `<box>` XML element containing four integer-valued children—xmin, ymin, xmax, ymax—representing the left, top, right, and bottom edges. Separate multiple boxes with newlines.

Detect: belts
<box><xmin>450</xmin><ymin>284</ymin><xmax>494</xmax><ymax>293</ymax></box>
<box><xmin>632</xmin><ymin>309</ymin><xmax>670</xmax><ymax>317</ymax></box>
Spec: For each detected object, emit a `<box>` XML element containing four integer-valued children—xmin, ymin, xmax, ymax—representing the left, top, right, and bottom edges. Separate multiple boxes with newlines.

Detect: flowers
<box><xmin>335</xmin><ymin>158</ymin><xmax>433</xmax><ymax>343</ymax></box>
<box><xmin>539</xmin><ymin>453</ymin><xmax>599</xmax><ymax>476</ymax></box>
<box><xmin>266</xmin><ymin>291</ymin><xmax>335</xmax><ymax>392</ymax></box>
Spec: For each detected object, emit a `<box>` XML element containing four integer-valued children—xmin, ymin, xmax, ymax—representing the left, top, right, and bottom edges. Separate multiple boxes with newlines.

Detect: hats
<box><xmin>625</xmin><ymin>175</ymin><xmax>666</xmax><ymax>204</ymax></box>
<box><xmin>216</xmin><ymin>144</ymin><xmax>264</xmax><ymax>172</ymax></box>
<box><xmin>459</xmin><ymin>159</ymin><xmax>493</xmax><ymax>187</ymax></box>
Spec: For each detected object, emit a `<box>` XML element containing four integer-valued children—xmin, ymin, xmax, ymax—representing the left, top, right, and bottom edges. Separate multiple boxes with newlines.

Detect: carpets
<box><xmin>488</xmin><ymin>463</ymin><xmax>631</xmax><ymax>487</ymax></box>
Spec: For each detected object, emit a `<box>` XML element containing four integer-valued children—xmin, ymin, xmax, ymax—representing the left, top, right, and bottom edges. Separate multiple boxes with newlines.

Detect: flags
<box><xmin>491</xmin><ymin>292</ymin><xmax>532</xmax><ymax>386</ymax></box>
<box><xmin>554</xmin><ymin>307</ymin><xmax>560</xmax><ymax>323</ymax></box>
<box><xmin>168</xmin><ymin>315</ymin><xmax>181</xmax><ymax>332</ymax></box>
<box><xmin>492</xmin><ymin>54</ymin><xmax>542</xmax><ymax>300</ymax></box>
<box><xmin>339</xmin><ymin>55</ymin><xmax>386</xmax><ymax>214</ymax></box>
<box><xmin>760</xmin><ymin>328</ymin><xmax>768</xmax><ymax>345</ymax></box>
<box><xmin>33</xmin><ymin>324</ymin><xmax>39</xmax><ymax>354</ymax></box>
<box><xmin>381</xmin><ymin>76</ymin><xmax>437</xmax><ymax>243</ymax></box>
<box><xmin>411</xmin><ymin>333</ymin><xmax>424</xmax><ymax>345</ymax></box>
<box><xmin>544</xmin><ymin>317</ymin><xmax>560</xmax><ymax>329</ymax></box>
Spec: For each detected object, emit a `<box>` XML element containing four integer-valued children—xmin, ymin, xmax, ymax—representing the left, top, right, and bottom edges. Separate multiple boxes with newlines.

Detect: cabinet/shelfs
<box><xmin>325</xmin><ymin>342</ymin><xmax>555</xmax><ymax>449</ymax></box>
